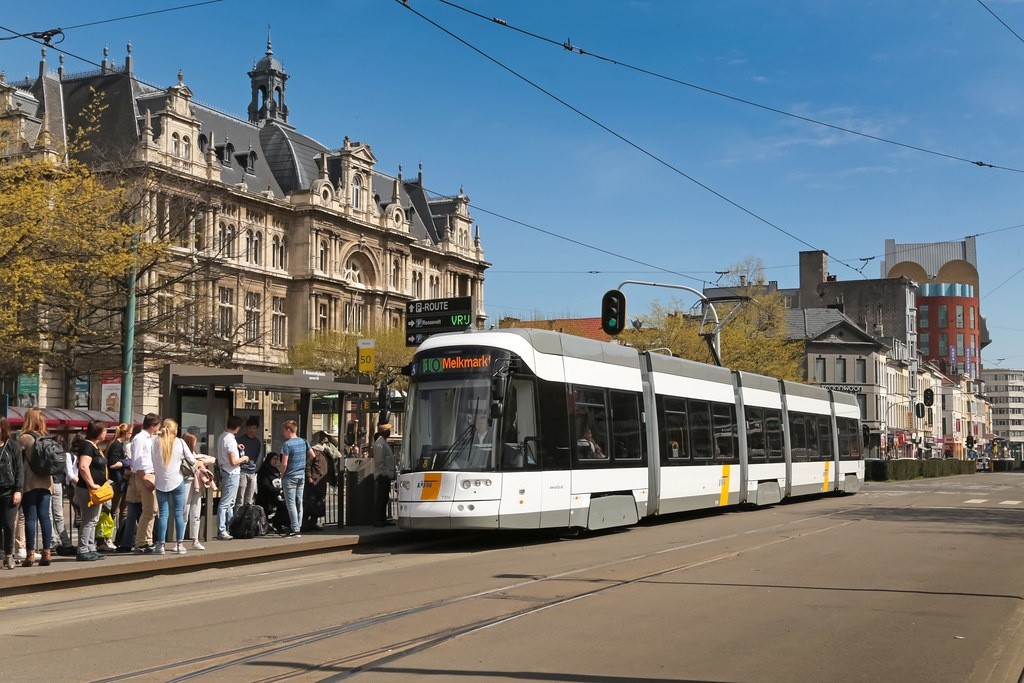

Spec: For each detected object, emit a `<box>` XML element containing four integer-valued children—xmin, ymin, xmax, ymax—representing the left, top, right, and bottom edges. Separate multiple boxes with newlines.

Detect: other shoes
<box><xmin>96</xmin><ymin>543</ymin><xmax>132</xmax><ymax>552</ymax></box>
<box><xmin>7</xmin><ymin>555</ymin><xmax>17</xmax><ymax>569</ymax></box>
<box><xmin>268</xmin><ymin>524</ymin><xmax>324</xmax><ymax>532</ymax></box>
<box><xmin>58</xmin><ymin>546</ymin><xmax>76</xmax><ymax>554</ymax></box>
<box><xmin>378</xmin><ymin>523</ymin><xmax>396</xmax><ymax>526</ymax></box>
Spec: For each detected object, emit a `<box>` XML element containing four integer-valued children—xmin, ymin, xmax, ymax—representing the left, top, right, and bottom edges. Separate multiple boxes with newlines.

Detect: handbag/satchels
<box><xmin>87</xmin><ymin>482</ymin><xmax>114</xmax><ymax>507</ymax></box>
<box><xmin>181</xmin><ymin>458</ymin><xmax>194</xmax><ymax>476</ymax></box>
<box><xmin>96</xmin><ymin>512</ymin><xmax>115</xmax><ymax>538</ymax></box>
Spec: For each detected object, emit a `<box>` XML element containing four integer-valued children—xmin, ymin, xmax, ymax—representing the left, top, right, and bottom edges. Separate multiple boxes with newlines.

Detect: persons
<box><xmin>19</xmin><ymin>407</ymin><xmax>53</xmax><ymax>566</ymax></box>
<box><xmin>172</xmin><ymin>433</ymin><xmax>205</xmax><ymax>552</ymax></box>
<box><xmin>217</xmin><ymin>415</ymin><xmax>249</xmax><ymax>540</ymax></box>
<box><xmin>18</xmin><ymin>393</ymin><xmax>37</xmax><ymax>407</ymax></box>
<box><xmin>106</xmin><ymin>422</ymin><xmax>159</xmax><ymax>552</ymax></box>
<box><xmin>64</xmin><ymin>419</ymin><xmax>117</xmax><ymax>560</ymax></box>
<box><xmin>281</xmin><ymin>420</ymin><xmax>339</xmax><ymax>538</ymax></box>
<box><xmin>235</xmin><ymin>418</ymin><xmax>261</xmax><ymax>516</ymax></box>
<box><xmin>0</xmin><ymin>415</ymin><xmax>25</xmax><ymax>569</ymax></box>
<box><xmin>252</xmin><ymin>452</ymin><xmax>291</xmax><ymax>532</ymax></box>
<box><xmin>943</xmin><ymin>445</ymin><xmax>952</xmax><ymax>460</ymax></box>
<box><xmin>373</xmin><ymin>424</ymin><xmax>397</xmax><ymax>527</ymax></box>
<box><xmin>580</xmin><ymin>427</ymin><xmax>605</xmax><ymax>458</ymax></box>
<box><xmin>475</xmin><ymin>417</ymin><xmax>491</xmax><ymax>444</ymax></box>
<box><xmin>188</xmin><ymin>425</ymin><xmax>200</xmax><ymax>454</ymax></box>
<box><xmin>13</xmin><ymin>507</ymin><xmax>42</xmax><ymax>559</ymax></box>
<box><xmin>669</xmin><ymin>441</ymin><xmax>682</xmax><ymax>458</ymax></box>
<box><xmin>130</xmin><ymin>413</ymin><xmax>162</xmax><ymax>553</ymax></box>
<box><xmin>147</xmin><ymin>418</ymin><xmax>195</xmax><ymax>555</ymax></box>
<box><xmin>49</xmin><ymin>435</ymin><xmax>78</xmax><ymax>556</ymax></box>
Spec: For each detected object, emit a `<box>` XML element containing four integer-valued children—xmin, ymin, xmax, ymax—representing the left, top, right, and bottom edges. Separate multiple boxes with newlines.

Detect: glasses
<box><xmin>273</xmin><ymin>457</ymin><xmax>279</xmax><ymax>460</ymax></box>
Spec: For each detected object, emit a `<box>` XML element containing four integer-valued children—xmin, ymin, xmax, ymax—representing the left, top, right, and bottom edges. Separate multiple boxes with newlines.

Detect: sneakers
<box><xmin>136</xmin><ymin>547</ymin><xmax>153</xmax><ymax>554</ymax></box>
<box><xmin>218</xmin><ymin>531</ymin><xmax>233</xmax><ymax>539</ymax></box>
<box><xmin>76</xmin><ymin>551</ymin><xmax>98</xmax><ymax>561</ymax></box>
<box><xmin>177</xmin><ymin>543</ymin><xmax>187</xmax><ymax>553</ymax></box>
<box><xmin>280</xmin><ymin>531</ymin><xmax>301</xmax><ymax>537</ymax></box>
<box><xmin>193</xmin><ymin>542</ymin><xmax>205</xmax><ymax>550</ymax></box>
<box><xmin>15</xmin><ymin>550</ymin><xmax>41</xmax><ymax>558</ymax></box>
<box><xmin>154</xmin><ymin>544</ymin><xmax>165</xmax><ymax>554</ymax></box>
<box><xmin>90</xmin><ymin>551</ymin><xmax>107</xmax><ymax>560</ymax></box>
<box><xmin>173</xmin><ymin>545</ymin><xmax>178</xmax><ymax>552</ymax></box>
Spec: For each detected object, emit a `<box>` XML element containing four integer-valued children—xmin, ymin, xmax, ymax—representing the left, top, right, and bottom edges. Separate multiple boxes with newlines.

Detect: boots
<box><xmin>21</xmin><ymin>550</ymin><xmax>34</xmax><ymax>567</ymax></box>
<box><xmin>39</xmin><ymin>549</ymin><xmax>51</xmax><ymax>566</ymax></box>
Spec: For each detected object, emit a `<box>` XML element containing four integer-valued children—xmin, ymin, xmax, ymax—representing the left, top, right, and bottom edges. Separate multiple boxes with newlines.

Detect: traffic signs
<box><xmin>405</xmin><ymin>328</ymin><xmax>475</xmax><ymax>348</ymax></box>
<box><xmin>405</xmin><ymin>296</ymin><xmax>474</xmax><ymax>318</ymax></box>
<box><xmin>406</xmin><ymin>313</ymin><xmax>477</xmax><ymax>331</ymax></box>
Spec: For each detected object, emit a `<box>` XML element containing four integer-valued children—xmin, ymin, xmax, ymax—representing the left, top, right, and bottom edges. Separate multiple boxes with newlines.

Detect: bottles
<box><xmin>240</xmin><ymin>450</ymin><xmax>245</xmax><ymax>463</ymax></box>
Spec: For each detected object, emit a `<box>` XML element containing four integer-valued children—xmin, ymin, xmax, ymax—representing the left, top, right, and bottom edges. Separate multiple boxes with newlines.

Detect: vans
<box><xmin>975</xmin><ymin>457</ymin><xmax>991</xmax><ymax>472</ymax></box>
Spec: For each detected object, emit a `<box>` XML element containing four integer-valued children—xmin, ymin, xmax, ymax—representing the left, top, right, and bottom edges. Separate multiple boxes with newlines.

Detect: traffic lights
<box><xmin>862</xmin><ymin>424</ymin><xmax>870</xmax><ymax>447</ymax></box>
<box><xmin>924</xmin><ymin>388</ymin><xmax>934</xmax><ymax>407</ymax></box>
<box><xmin>967</xmin><ymin>436</ymin><xmax>973</xmax><ymax>448</ymax></box>
<box><xmin>601</xmin><ymin>290</ymin><xmax>627</xmax><ymax>335</ymax></box>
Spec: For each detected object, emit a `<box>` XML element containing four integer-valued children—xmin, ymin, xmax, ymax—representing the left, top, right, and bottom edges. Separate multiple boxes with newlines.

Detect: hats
<box><xmin>379</xmin><ymin>424</ymin><xmax>392</xmax><ymax>432</ymax></box>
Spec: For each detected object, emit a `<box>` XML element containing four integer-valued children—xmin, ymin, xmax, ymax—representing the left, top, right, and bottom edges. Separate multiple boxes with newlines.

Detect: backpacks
<box><xmin>229</xmin><ymin>505</ymin><xmax>268</xmax><ymax>537</ymax></box>
<box><xmin>0</xmin><ymin>447</ymin><xmax>14</xmax><ymax>487</ymax></box>
<box><xmin>29</xmin><ymin>431</ymin><xmax>67</xmax><ymax>476</ymax></box>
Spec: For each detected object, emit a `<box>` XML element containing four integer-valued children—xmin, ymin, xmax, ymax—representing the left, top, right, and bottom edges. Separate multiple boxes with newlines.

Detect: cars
<box><xmin>864</xmin><ymin>457</ymin><xmax>960</xmax><ymax>461</ymax></box>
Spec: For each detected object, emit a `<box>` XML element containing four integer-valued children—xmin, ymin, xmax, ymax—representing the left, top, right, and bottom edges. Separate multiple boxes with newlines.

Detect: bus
<box><xmin>377</xmin><ymin>327</ymin><xmax>865</xmax><ymax>536</ymax></box>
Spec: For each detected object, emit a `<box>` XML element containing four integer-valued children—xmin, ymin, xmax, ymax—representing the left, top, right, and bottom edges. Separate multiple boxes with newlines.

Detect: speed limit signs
<box><xmin>359</xmin><ymin>347</ymin><xmax>375</xmax><ymax>372</ymax></box>
